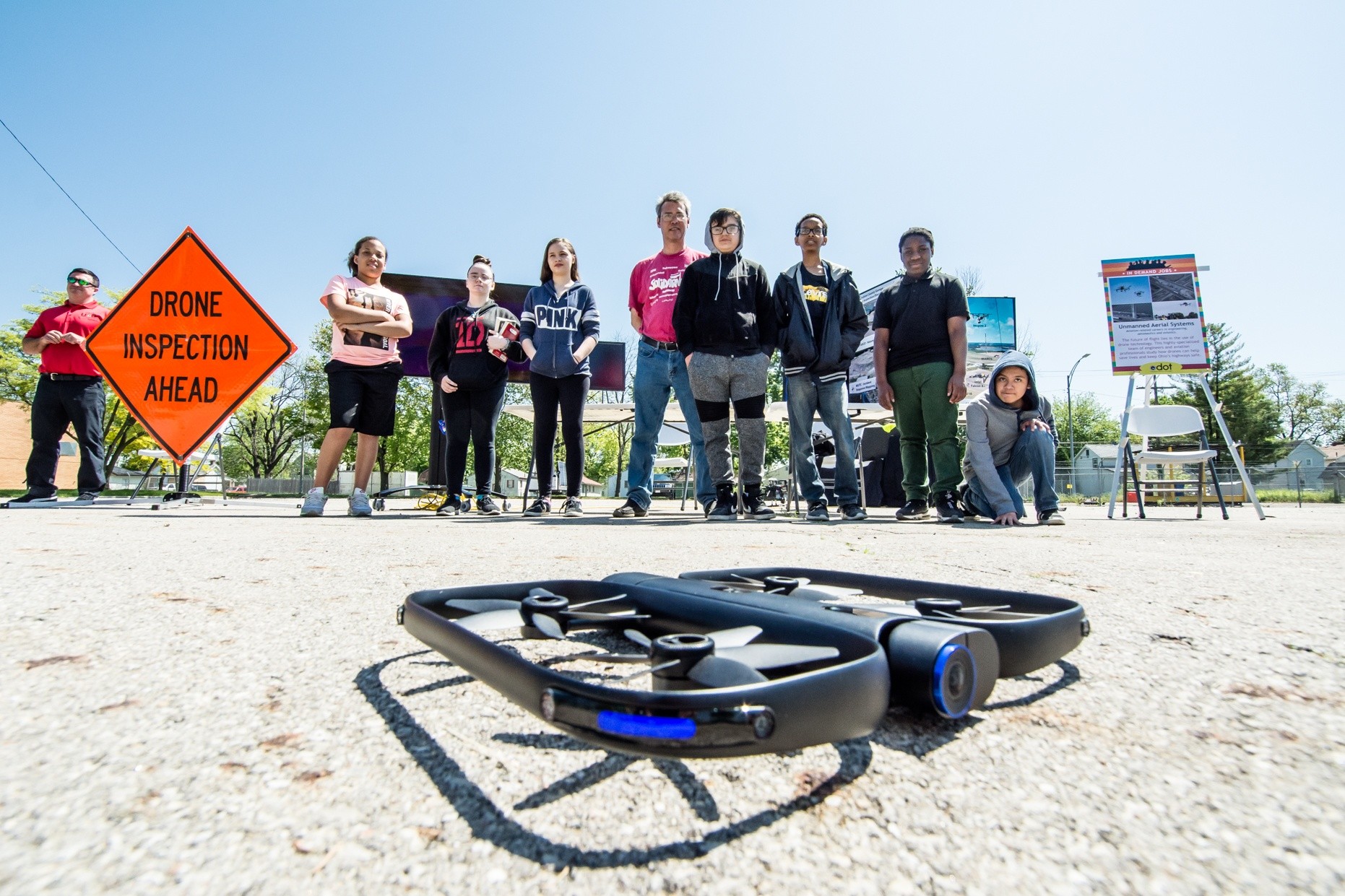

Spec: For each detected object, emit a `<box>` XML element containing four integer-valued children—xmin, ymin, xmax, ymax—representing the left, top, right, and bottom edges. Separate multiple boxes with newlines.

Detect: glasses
<box><xmin>68</xmin><ymin>277</ymin><xmax>97</xmax><ymax>288</ymax></box>
<box><xmin>797</xmin><ymin>228</ymin><xmax>825</xmax><ymax>237</ymax></box>
<box><xmin>468</xmin><ymin>274</ymin><xmax>492</xmax><ymax>281</ymax></box>
<box><xmin>663</xmin><ymin>214</ymin><xmax>687</xmax><ymax>222</ymax></box>
<box><xmin>710</xmin><ymin>225</ymin><xmax>740</xmax><ymax>234</ymax></box>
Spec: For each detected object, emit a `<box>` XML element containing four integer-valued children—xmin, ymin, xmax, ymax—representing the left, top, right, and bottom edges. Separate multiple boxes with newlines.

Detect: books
<box><xmin>489</xmin><ymin>317</ymin><xmax>520</xmax><ymax>362</ymax></box>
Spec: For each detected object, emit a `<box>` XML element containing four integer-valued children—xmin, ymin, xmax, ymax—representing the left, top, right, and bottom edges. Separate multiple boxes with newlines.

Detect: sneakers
<box><xmin>1037</xmin><ymin>507</ymin><xmax>1067</xmax><ymax>525</ymax></box>
<box><xmin>806</xmin><ymin>500</ymin><xmax>829</xmax><ymax>521</ymax></box>
<box><xmin>477</xmin><ymin>497</ymin><xmax>502</xmax><ymax>515</ymax></box>
<box><xmin>839</xmin><ymin>504</ymin><xmax>868</xmax><ymax>520</ymax></box>
<box><xmin>524</xmin><ymin>495</ymin><xmax>551</xmax><ymax>516</ymax></box>
<box><xmin>559</xmin><ymin>496</ymin><xmax>583</xmax><ymax>517</ymax></box>
<box><xmin>958</xmin><ymin>483</ymin><xmax>982</xmax><ymax>520</ymax></box>
<box><xmin>741</xmin><ymin>488</ymin><xmax>776</xmax><ymax>519</ymax></box>
<box><xmin>300</xmin><ymin>486</ymin><xmax>328</xmax><ymax>516</ymax></box>
<box><xmin>934</xmin><ymin>490</ymin><xmax>965</xmax><ymax>523</ymax></box>
<box><xmin>436</xmin><ymin>493</ymin><xmax>461</xmax><ymax>516</ymax></box>
<box><xmin>347</xmin><ymin>487</ymin><xmax>372</xmax><ymax>516</ymax></box>
<box><xmin>707</xmin><ymin>489</ymin><xmax>738</xmax><ymax>520</ymax></box>
<box><xmin>896</xmin><ymin>499</ymin><xmax>930</xmax><ymax>521</ymax></box>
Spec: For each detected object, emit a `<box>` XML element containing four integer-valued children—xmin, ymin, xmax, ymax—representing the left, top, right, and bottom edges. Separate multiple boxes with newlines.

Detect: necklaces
<box><xmin>468</xmin><ymin>301</ymin><xmax>482</xmax><ymax>318</ymax></box>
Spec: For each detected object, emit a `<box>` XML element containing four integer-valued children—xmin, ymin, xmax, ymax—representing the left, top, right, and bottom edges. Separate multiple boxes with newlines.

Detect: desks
<box><xmin>500</xmin><ymin>403</ymin><xmax>768</xmax><ymax>515</ymax></box>
<box><xmin>780</xmin><ymin>417</ymin><xmax>966</xmax><ymax>445</ymax></box>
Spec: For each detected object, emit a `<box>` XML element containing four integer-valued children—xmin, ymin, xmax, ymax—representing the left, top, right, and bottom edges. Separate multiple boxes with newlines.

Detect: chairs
<box><xmin>1120</xmin><ymin>404</ymin><xmax>1229</xmax><ymax>519</ymax></box>
<box><xmin>786</xmin><ymin>410</ymin><xmax>865</xmax><ymax>515</ymax></box>
<box><xmin>651</xmin><ymin>422</ymin><xmax>698</xmax><ymax>511</ymax></box>
<box><xmin>125</xmin><ymin>412</ymin><xmax>234</xmax><ymax>507</ymax></box>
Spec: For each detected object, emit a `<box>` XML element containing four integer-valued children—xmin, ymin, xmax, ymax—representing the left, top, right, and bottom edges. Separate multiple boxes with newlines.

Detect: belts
<box><xmin>40</xmin><ymin>372</ymin><xmax>102</xmax><ymax>382</ymax></box>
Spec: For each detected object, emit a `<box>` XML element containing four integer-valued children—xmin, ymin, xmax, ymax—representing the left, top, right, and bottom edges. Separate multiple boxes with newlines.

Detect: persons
<box><xmin>962</xmin><ymin>350</ymin><xmax>1065</xmax><ymax>525</ymax></box>
<box><xmin>613</xmin><ymin>192</ymin><xmax>718</xmax><ymax>518</ymax></box>
<box><xmin>521</xmin><ymin>237</ymin><xmax>601</xmax><ymax>515</ymax></box>
<box><xmin>9</xmin><ymin>268</ymin><xmax>112</xmax><ymax>503</ymax></box>
<box><xmin>300</xmin><ymin>237</ymin><xmax>412</xmax><ymax>516</ymax></box>
<box><xmin>671</xmin><ymin>209</ymin><xmax>780</xmax><ymax>521</ymax></box>
<box><xmin>872</xmin><ymin>229</ymin><xmax>971</xmax><ymax>523</ymax></box>
<box><xmin>428</xmin><ymin>254</ymin><xmax>528</xmax><ymax>516</ymax></box>
<box><xmin>771</xmin><ymin>213</ymin><xmax>869</xmax><ymax>521</ymax></box>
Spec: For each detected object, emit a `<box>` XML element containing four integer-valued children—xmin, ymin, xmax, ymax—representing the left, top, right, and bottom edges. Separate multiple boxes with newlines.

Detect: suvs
<box><xmin>650</xmin><ymin>474</ymin><xmax>676</xmax><ymax>500</ymax></box>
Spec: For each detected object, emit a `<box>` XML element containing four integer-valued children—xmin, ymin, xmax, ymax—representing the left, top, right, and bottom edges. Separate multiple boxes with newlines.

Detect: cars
<box><xmin>191</xmin><ymin>485</ymin><xmax>207</xmax><ymax>492</ymax></box>
<box><xmin>226</xmin><ymin>485</ymin><xmax>247</xmax><ymax>494</ymax></box>
<box><xmin>162</xmin><ymin>483</ymin><xmax>176</xmax><ymax>492</ymax></box>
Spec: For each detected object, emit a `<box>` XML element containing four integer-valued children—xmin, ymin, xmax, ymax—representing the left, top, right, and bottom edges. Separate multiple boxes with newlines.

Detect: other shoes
<box><xmin>705</xmin><ymin>501</ymin><xmax>717</xmax><ymax>518</ymax></box>
<box><xmin>75</xmin><ymin>491</ymin><xmax>99</xmax><ymax>501</ymax></box>
<box><xmin>612</xmin><ymin>497</ymin><xmax>649</xmax><ymax>518</ymax></box>
<box><xmin>7</xmin><ymin>490</ymin><xmax>58</xmax><ymax>502</ymax></box>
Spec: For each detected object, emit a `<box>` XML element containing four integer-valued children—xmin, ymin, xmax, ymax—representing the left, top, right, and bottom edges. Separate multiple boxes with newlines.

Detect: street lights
<box><xmin>284</xmin><ymin>361</ymin><xmax>307</xmax><ymax>493</ymax></box>
<box><xmin>1067</xmin><ymin>353</ymin><xmax>1091</xmax><ymax>496</ymax></box>
